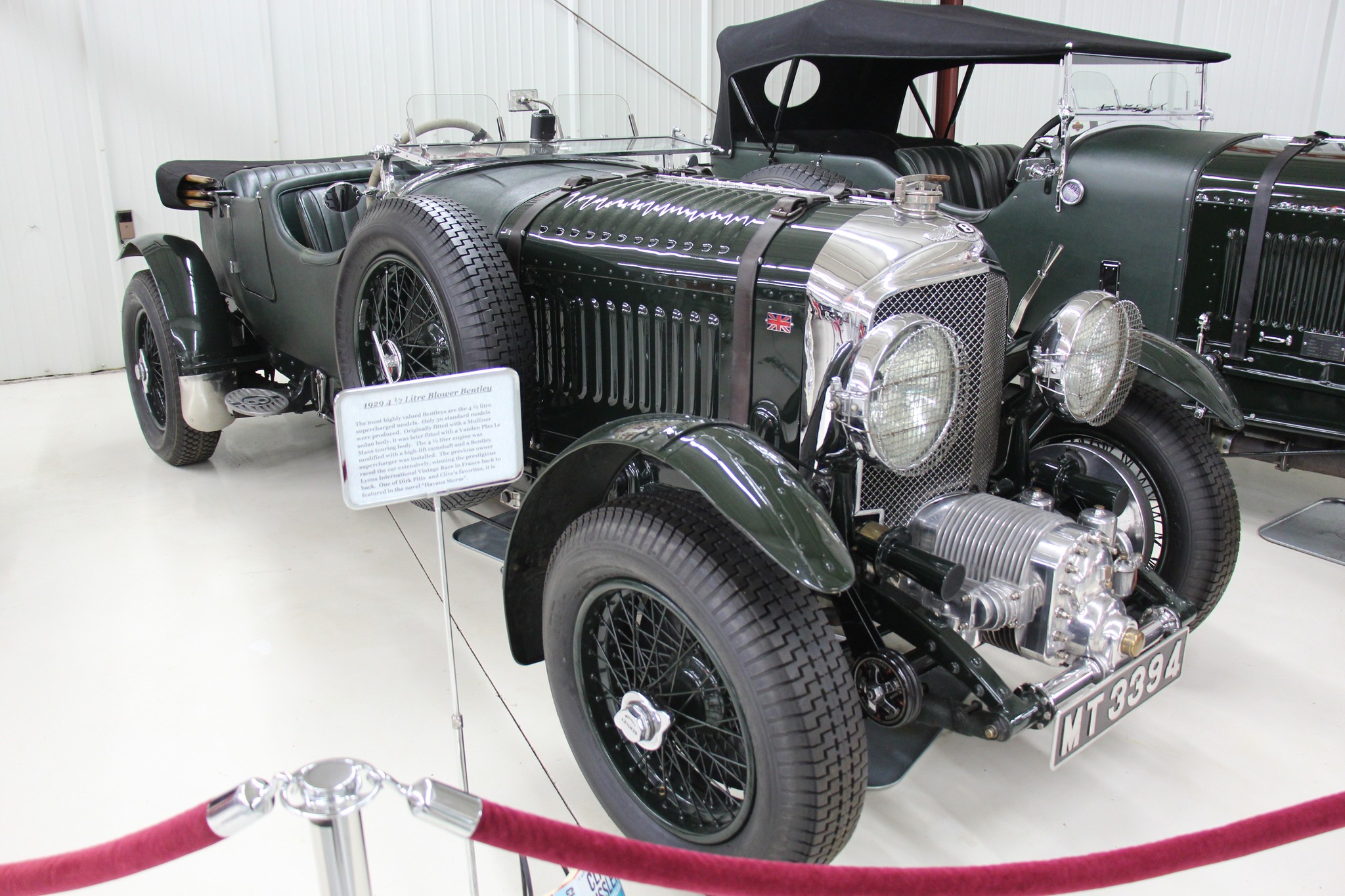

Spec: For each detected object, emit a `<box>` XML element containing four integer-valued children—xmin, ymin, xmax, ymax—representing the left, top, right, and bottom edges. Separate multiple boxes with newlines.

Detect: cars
<box><xmin>712</xmin><ymin>1</ymin><xmax>1344</xmax><ymax>472</ymax></box>
<box><xmin>113</xmin><ymin>107</ymin><xmax>1245</xmax><ymax>864</ymax></box>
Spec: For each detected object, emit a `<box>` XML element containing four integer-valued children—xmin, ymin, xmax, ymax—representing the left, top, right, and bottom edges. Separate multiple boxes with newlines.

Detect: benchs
<box><xmin>735</xmin><ymin>130</ymin><xmax>900</xmax><ymax>169</ymax></box>
<box><xmin>295</xmin><ymin>174</ymin><xmax>406</xmax><ymax>252</ymax></box>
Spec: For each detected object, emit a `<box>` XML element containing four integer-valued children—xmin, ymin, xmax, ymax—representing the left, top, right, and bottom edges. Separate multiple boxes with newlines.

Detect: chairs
<box><xmin>959</xmin><ymin>143</ymin><xmax>1034</xmax><ymax>208</ymax></box>
<box><xmin>896</xmin><ymin>146</ymin><xmax>985</xmax><ymax>211</ymax></box>
